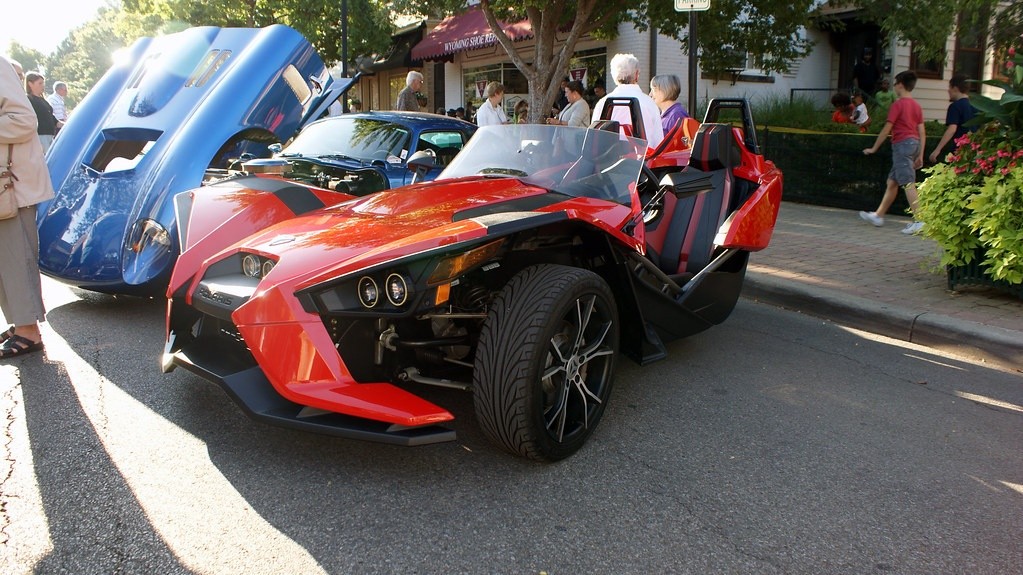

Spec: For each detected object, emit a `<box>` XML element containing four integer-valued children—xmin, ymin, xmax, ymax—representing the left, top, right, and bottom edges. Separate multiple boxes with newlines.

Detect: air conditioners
<box><xmin>706</xmin><ymin>43</ymin><xmax>748</xmax><ymax>71</ymax></box>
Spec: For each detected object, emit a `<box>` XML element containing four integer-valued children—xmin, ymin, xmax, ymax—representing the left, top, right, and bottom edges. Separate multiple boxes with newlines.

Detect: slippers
<box><xmin>0</xmin><ymin>335</ymin><xmax>43</xmax><ymax>358</ymax></box>
<box><xmin>0</xmin><ymin>325</ymin><xmax>16</xmax><ymax>344</ymax></box>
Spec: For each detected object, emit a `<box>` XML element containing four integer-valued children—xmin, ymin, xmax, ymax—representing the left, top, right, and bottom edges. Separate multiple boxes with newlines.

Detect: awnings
<box><xmin>345</xmin><ymin>4</ymin><xmax>575</xmax><ymax>78</ymax></box>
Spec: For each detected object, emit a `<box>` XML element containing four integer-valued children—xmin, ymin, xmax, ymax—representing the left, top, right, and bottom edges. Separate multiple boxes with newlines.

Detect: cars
<box><xmin>36</xmin><ymin>24</ymin><xmax>478</xmax><ymax>299</ymax></box>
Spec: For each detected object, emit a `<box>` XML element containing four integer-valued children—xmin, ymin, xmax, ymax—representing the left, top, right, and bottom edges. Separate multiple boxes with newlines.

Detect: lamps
<box><xmin>348</xmin><ymin>99</ymin><xmax>361</xmax><ymax>105</ymax></box>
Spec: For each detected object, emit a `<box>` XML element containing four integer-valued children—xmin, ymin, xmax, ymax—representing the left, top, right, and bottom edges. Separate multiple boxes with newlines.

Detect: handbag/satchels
<box><xmin>0</xmin><ymin>171</ymin><xmax>18</xmax><ymax>221</ymax></box>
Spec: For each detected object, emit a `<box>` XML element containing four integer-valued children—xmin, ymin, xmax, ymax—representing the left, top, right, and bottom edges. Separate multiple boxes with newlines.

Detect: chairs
<box><xmin>643</xmin><ymin>121</ymin><xmax>740</xmax><ymax>286</ymax></box>
<box><xmin>557</xmin><ymin>120</ymin><xmax>619</xmax><ymax>186</ymax></box>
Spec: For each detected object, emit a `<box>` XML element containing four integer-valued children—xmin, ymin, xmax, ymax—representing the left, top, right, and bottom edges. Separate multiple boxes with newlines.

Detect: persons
<box><xmin>849</xmin><ymin>90</ymin><xmax>867</xmax><ymax>131</ymax></box>
<box><xmin>0</xmin><ymin>57</ymin><xmax>54</xmax><ymax>357</ymax></box>
<box><xmin>831</xmin><ymin>92</ymin><xmax>872</xmax><ymax>132</ymax></box>
<box><xmin>929</xmin><ymin>73</ymin><xmax>982</xmax><ymax>162</ymax></box>
<box><xmin>859</xmin><ymin>70</ymin><xmax>926</xmax><ymax>234</ymax></box>
<box><xmin>47</xmin><ymin>81</ymin><xmax>67</xmax><ymax>137</ymax></box>
<box><xmin>24</xmin><ymin>72</ymin><xmax>64</xmax><ymax>154</ymax></box>
<box><xmin>350</xmin><ymin>54</ymin><xmax>688</xmax><ymax>149</ymax></box>
<box><xmin>875</xmin><ymin>78</ymin><xmax>897</xmax><ymax>110</ymax></box>
<box><xmin>10</xmin><ymin>60</ymin><xmax>23</xmax><ymax>80</ymax></box>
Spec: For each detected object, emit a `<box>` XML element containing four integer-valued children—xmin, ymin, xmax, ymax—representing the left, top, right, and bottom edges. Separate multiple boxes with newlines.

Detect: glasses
<box><xmin>893</xmin><ymin>82</ymin><xmax>900</xmax><ymax>87</ymax></box>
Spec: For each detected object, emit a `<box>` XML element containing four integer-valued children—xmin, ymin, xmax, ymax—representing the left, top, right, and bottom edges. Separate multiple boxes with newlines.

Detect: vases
<box><xmin>945</xmin><ymin>228</ymin><xmax>1023</xmax><ymax>302</ymax></box>
<box><xmin>417</xmin><ymin>98</ymin><xmax>427</xmax><ymax>108</ymax></box>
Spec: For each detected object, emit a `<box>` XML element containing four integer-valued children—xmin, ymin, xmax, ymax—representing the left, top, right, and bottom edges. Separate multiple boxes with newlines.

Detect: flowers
<box><xmin>416</xmin><ymin>92</ymin><xmax>424</xmax><ymax>98</ymax></box>
<box><xmin>902</xmin><ymin>118</ymin><xmax>1023</xmax><ymax>285</ymax></box>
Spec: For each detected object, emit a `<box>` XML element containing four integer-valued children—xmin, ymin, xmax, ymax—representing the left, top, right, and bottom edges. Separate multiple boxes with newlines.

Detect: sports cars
<box><xmin>159</xmin><ymin>98</ymin><xmax>784</xmax><ymax>465</ymax></box>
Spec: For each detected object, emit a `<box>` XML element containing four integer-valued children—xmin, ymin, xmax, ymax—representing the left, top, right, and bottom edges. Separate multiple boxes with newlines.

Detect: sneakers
<box><xmin>902</xmin><ymin>221</ymin><xmax>926</xmax><ymax>234</ymax></box>
<box><xmin>860</xmin><ymin>211</ymin><xmax>884</xmax><ymax>227</ymax></box>
